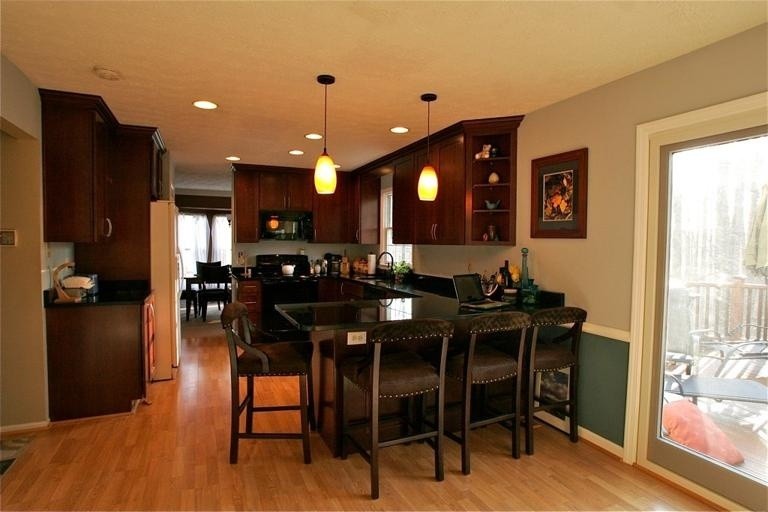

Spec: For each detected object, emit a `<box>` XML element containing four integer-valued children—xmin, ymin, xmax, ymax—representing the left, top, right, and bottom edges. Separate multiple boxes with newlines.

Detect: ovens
<box><xmin>260</xmin><ymin>277</ymin><xmax>317</xmax><ymax>342</ymax></box>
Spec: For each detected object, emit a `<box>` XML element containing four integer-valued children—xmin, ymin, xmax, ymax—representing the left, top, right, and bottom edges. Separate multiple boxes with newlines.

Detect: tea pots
<box><xmin>280</xmin><ymin>263</ymin><xmax>297</xmax><ymax>275</ymax></box>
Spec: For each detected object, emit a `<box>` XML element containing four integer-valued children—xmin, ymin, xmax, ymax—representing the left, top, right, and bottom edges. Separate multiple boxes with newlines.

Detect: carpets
<box><xmin>0</xmin><ymin>433</ymin><xmax>36</xmax><ymax>481</ymax></box>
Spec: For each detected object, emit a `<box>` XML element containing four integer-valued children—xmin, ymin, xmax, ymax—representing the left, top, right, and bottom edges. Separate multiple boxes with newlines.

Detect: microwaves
<box><xmin>260</xmin><ymin>211</ymin><xmax>313</xmax><ymax>243</ymax></box>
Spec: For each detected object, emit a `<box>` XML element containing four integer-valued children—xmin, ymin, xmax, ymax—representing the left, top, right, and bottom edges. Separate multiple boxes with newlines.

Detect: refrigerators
<box><xmin>149</xmin><ymin>203</ymin><xmax>185</xmax><ymax>381</ymax></box>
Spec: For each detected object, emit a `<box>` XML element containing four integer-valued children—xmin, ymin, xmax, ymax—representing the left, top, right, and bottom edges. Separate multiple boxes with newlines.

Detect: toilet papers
<box><xmin>368</xmin><ymin>254</ymin><xmax>376</xmax><ymax>275</ymax></box>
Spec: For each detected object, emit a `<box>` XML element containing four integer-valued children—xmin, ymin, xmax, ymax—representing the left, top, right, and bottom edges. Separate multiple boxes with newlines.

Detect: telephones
<box><xmin>484</xmin><ymin>271</ymin><xmax>499</xmax><ymax>296</ymax></box>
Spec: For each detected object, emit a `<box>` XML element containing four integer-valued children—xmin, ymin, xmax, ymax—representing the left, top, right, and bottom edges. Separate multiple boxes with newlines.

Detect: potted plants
<box><xmin>394</xmin><ymin>261</ymin><xmax>414</xmax><ymax>284</ymax></box>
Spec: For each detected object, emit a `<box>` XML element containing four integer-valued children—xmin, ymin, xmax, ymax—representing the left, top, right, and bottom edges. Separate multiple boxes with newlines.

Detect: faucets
<box><xmin>376</xmin><ymin>251</ymin><xmax>393</xmax><ymax>269</ymax></box>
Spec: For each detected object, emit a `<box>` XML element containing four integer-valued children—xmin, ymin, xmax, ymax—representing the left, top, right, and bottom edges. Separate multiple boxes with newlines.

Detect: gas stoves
<box><xmin>254</xmin><ymin>269</ymin><xmax>318</xmax><ymax>283</ymax></box>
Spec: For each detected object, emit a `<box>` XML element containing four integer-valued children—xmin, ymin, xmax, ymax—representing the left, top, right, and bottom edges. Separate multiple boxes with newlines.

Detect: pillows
<box><xmin>661</xmin><ymin>398</ymin><xmax>744</xmax><ymax>465</ymax></box>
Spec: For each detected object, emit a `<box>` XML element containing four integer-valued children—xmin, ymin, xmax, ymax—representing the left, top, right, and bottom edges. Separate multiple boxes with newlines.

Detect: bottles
<box><xmin>310</xmin><ymin>259</ymin><xmax>327</xmax><ymax>276</ymax></box>
<box><xmin>487</xmin><ymin>223</ymin><xmax>497</xmax><ymax>241</ymax></box>
<box><xmin>480</xmin><ymin>246</ymin><xmax>540</xmax><ymax>305</ymax></box>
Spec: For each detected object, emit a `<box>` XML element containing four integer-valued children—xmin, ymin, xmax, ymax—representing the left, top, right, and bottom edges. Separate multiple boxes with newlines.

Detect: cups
<box><xmin>89</xmin><ymin>274</ymin><xmax>98</xmax><ymax>295</ymax></box>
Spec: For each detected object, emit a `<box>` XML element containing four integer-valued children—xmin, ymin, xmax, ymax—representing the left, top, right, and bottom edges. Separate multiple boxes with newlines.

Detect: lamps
<box><xmin>417</xmin><ymin>94</ymin><xmax>438</xmax><ymax>201</ymax></box>
<box><xmin>314</xmin><ymin>75</ymin><xmax>337</xmax><ymax>194</ymax></box>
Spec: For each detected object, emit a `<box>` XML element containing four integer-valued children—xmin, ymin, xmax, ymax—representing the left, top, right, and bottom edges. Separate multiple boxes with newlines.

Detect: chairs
<box><xmin>180</xmin><ymin>261</ymin><xmax>232</xmax><ymax>322</ymax></box>
<box><xmin>664</xmin><ymin>323</ymin><xmax>768</xmax><ymax>404</ymax></box>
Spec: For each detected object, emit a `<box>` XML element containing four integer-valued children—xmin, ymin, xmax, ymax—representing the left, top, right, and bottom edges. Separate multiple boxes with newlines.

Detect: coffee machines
<box><xmin>324</xmin><ymin>252</ymin><xmax>341</xmax><ymax>278</ymax></box>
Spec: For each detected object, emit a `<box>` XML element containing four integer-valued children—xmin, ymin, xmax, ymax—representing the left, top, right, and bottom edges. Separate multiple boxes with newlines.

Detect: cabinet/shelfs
<box><xmin>232</xmin><ymin>279</ymin><xmax>363</xmax><ymax>337</ymax></box>
<box><xmin>232</xmin><ymin>115</ymin><xmax>525</xmax><ymax>246</ymax></box>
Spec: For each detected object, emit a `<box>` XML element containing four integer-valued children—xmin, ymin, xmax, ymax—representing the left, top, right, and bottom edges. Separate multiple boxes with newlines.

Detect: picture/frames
<box><xmin>530</xmin><ymin>147</ymin><xmax>588</xmax><ymax>238</ymax></box>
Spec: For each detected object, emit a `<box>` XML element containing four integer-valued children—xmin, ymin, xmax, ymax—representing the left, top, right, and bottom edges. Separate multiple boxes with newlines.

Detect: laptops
<box><xmin>452</xmin><ymin>273</ymin><xmax>511</xmax><ymax>310</ymax></box>
